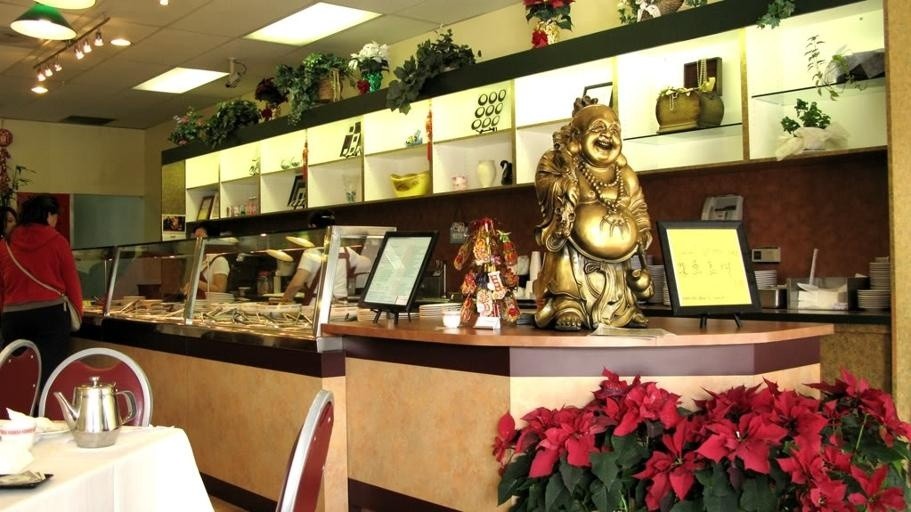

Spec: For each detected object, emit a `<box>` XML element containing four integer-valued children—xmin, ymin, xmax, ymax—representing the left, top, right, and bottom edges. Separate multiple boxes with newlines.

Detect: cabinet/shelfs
<box><xmin>161</xmin><ymin>0</ymin><xmax>887</xmax><ymax>244</ymax></box>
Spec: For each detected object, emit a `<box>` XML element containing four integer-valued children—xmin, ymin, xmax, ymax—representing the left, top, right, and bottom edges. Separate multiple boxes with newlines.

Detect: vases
<box><xmin>363</xmin><ymin>71</ymin><xmax>383</xmax><ymax>91</ymax></box>
<box><xmin>177</xmin><ymin>138</ymin><xmax>188</xmax><ymax>145</ymax></box>
<box><xmin>535</xmin><ymin>22</ymin><xmax>560</xmax><ymax>45</ymax></box>
<box><xmin>271</xmin><ymin>107</ymin><xmax>281</xmax><ymax>120</ymax></box>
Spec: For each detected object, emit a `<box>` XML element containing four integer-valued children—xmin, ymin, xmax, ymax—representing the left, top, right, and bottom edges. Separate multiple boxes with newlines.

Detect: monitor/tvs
<box><xmin>359</xmin><ymin>230</ymin><xmax>439</xmax><ymax>324</ymax></box>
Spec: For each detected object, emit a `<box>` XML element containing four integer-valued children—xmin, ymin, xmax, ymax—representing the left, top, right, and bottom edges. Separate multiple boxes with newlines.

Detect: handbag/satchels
<box><xmin>64</xmin><ymin>295</ymin><xmax>84</xmax><ymax>333</ymax></box>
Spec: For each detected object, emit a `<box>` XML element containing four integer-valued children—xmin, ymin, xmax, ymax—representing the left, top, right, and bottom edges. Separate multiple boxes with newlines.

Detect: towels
<box><xmin>6</xmin><ymin>406</ymin><xmax>54</xmax><ymax>433</ymax></box>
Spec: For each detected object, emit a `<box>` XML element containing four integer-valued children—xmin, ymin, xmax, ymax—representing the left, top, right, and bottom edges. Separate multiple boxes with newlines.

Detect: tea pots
<box><xmin>53</xmin><ymin>376</ymin><xmax>138</xmax><ymax>449</ymax></box>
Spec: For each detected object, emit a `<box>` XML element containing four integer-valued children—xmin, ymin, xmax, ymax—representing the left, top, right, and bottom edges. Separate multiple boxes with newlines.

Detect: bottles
<box><xmin>227</xmin><ymin>196</ymin><xmax>257</xmax><ymax>218</ymax></box>
<box><xmin>273</xmin><ymin>270</ymin><xmax>281</xmax><ymax>293</ymax></box>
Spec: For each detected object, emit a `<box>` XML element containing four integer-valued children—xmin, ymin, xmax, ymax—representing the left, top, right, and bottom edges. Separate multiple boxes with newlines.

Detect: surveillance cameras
<box><xmin>224</xmin><ymin>71</ymin><xmax>242</xmax><ymax>88</ymax></box>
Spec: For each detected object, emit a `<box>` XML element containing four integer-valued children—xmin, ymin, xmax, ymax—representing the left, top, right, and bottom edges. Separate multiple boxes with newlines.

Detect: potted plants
<box><xmin>779</xmin><ymin>97</ymin><xmax>831</xmax><ymax>150</ymax></box>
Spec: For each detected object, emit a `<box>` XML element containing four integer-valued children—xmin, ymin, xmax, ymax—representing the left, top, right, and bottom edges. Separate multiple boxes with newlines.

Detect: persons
<box><xmin>181</xmin><ymin>227</ymin><xmax>230</xmax><ymax>300</ymax></box>
<box><xmin>0</xmin><ymin>206</ymin><xmax>18</xmax><ymax>240</ymax></box>
<box><xmin>283</xmin><ymin>228</ymin><xmax>362</xmax><ymax>307</ymax></box>
<box><xmin>535</xmin><ymin>95</ymin><xmax>656</xmax><ymax>330</ymax></box>
<box><xmin>0</xmin><ymin>191</ymin><xmax>83</xmax><ymax>399</ymax></box>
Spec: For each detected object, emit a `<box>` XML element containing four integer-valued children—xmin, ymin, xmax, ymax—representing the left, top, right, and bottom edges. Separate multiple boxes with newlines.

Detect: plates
<box><xmin>858</xmin><ymin>256</ymin><xmax>891</xmax><ymax>313</ymax></box>
<box><xmin>0</xmin><ymin>473</ymin><xmax>55</xmax><ymax>490</ymax></box>
<box><xmin>356</xmin><ymin>302</ymin><xmax>462</xmax><ymax>322</ymax></box>
<box><xmin>632</xmin><ymin>253</ymin><xmax>671</xmax><ymax>307</ymax></box>
<box><xmin>38</xmin><ymin>421</ymin><xmax>72</xmax><ymax>440</ymax></box>
<box><xmin>112</xmin><ymin>295</ymin><xmax>162</xmax><ymax>304</ymax></box>
<box><xmin>753</xmin><ymin>269</ymin><xmax>779</xmax><ymax>288</ymax></box>
<box><xmin>184</xmin><ymin>292</ymin><xmax>234</xmax><ymax>309</ymax></box>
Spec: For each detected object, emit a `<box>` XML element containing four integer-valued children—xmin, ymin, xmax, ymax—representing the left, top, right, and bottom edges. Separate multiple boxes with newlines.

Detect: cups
<box><xmin>443</xmin><ymin>311</ymin><xmax>461</xmax><ymax>328</ymax></box>
<box><xmin>238</xmin><ymin>287</ymin><xmax>251</xmax><ymax>297</ymax></box>
<box><xmin>525</xmin><ymin>250</ymin><xmax>541</xmax><ymax>298</ymax></box>
<box><xmin>0</xmin><ymin>419</ymin><xmax>40</xmax><ymax>452</ymax></box>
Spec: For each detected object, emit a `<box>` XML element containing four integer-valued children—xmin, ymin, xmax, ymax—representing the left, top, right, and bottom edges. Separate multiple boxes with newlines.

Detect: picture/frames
<box><xmin>195</xmin><ymin>196</ymin><xmax>213</xmax><ymax>221</ymax></box>
<box><xmin>357</xmin><ymin>231</ymin><xmax>435</xmax><ymax>312</ymax></box>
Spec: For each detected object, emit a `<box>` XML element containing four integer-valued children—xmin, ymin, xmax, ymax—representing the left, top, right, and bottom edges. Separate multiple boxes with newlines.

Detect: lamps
<box><xmin>9</xmin><ymin>2</ymin><xmax>77</xmax><ymax>41</ymax></box>
<box><xmin>33</xmin><ymin>18</ymin><xmax>110</xmax><ymax>82</ymax></box>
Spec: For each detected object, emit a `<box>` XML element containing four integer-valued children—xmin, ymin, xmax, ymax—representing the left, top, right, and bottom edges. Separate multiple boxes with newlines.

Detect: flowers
<box><xmin>616</xmin><ymin>0</ymin><xmax>708</xmax><ymax>25</ymax></box>
<box><xmin>492</xmin><ymin>368</ymin><xmax>911</xmax><ymax>512</ymax></box>
<box><xmin>167</xmin><ymin>105</ymin><xmax>207</xmax><ymax>142</ymax></box>
<box><xmin>348</xmin><ymin>41</ymin><xmax>389</xmax><ymax>75</ymax></box>
<box><xmin>521</xmin><ymin>0</ymin><xmax>576</xmax><ymax>32</ymax></box>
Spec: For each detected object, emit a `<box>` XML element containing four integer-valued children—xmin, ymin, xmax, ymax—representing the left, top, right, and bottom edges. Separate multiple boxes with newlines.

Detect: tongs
<box><xmin>89</xmin><ymin>295</ymin><xmax>106</xmax><ymax>307</ymax></box>
<box><xmin>120</xmin><ymin>300</ymin><xmax>313</xmax><ymax>328</ymax></box>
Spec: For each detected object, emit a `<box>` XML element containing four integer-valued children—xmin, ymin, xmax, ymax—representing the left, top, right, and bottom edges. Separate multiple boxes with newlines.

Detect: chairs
<box><xmin>276</xmin><ymin>389</ymin><xmax>335</xmax><ymax>512</ymax></box>
<box><xmin>1</xmin><ymin>338</ymin><xmax>153</xmax><ymax>427</ymax></box>
